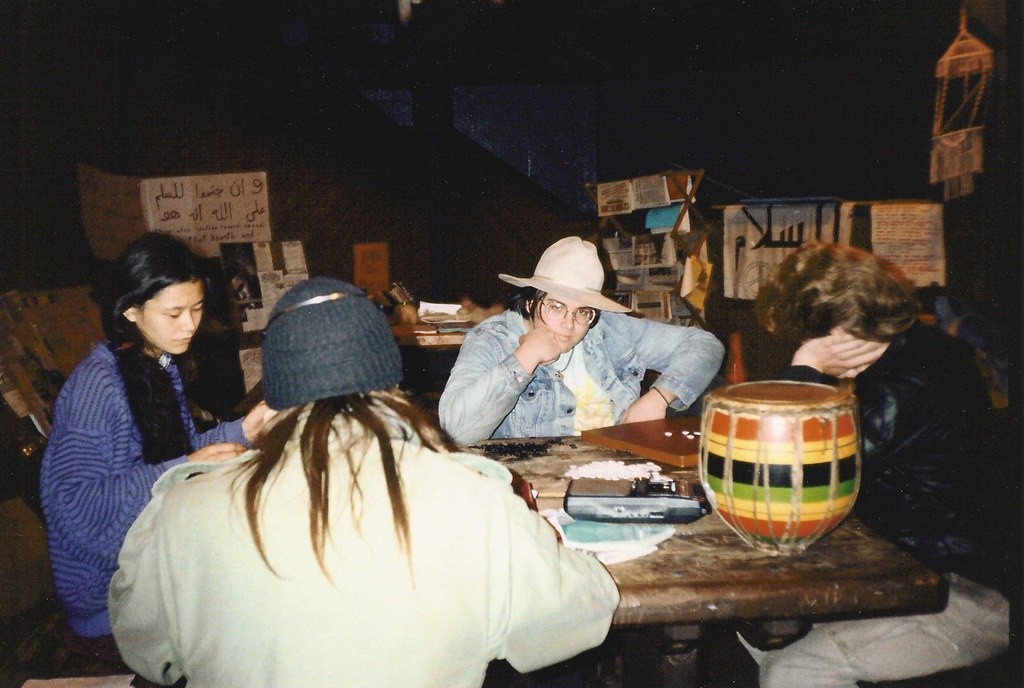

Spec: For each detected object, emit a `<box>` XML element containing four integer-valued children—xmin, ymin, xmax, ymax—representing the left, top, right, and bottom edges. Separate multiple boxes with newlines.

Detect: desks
<box><xmin>385</xmin><ymin>315</ymin><xmax>464</xmax><ymax>347</ymax></box>
<box><xmin>475</xmin><ymin>435</ymin><xmax>949</xmax><ymax>688</ymax></box>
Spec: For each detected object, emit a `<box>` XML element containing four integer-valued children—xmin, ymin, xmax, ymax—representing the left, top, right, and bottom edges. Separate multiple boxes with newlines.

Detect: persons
<box><xmin>736</xmin><ymin>241</ymin><xmax>1023</xmax><ymax>688</ymax></box>
<box><xmin>437</xmin><ymin>236</ymin><xmax>727</xmax><ymax>447</ymax></box>
<box><xmin>108</xmin><ymin>274</ymin><xmax>620</xmax><ymax>688</ymax></box>
<box><xmin>39</xmin><ymin>230</ymin><xmax>280</xmax><ymax>640</ymax></box>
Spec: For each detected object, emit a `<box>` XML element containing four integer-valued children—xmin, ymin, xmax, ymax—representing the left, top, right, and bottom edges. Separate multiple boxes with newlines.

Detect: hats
<box><xmin>498</xmin><ymin>237</ymin><xmax>633</xmax><ymax>313</ymax></box>
<box><xmin>260</xmin><ymin>278</ymin><xmax>402</xmax><ymax>411</ymax></box>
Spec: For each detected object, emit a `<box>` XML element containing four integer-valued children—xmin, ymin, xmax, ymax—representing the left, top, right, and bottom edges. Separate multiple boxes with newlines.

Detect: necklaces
<box><xmin>554</xmin><ymin>347</ymin><xmax>574</xmax><ymax>379</ymax></box>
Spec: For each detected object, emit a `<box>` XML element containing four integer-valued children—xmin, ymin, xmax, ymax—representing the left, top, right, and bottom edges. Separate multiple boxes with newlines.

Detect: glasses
<box><xmin>535</xmin><ymin>298</ymin><xmax>596</xmax><ymax>326</ymax></box>
